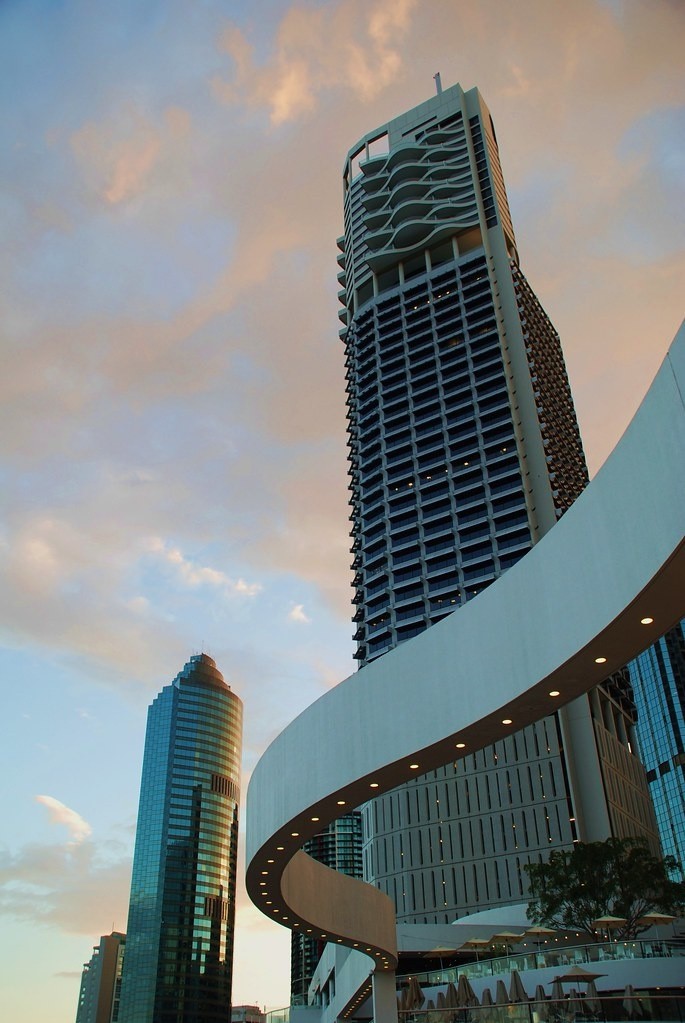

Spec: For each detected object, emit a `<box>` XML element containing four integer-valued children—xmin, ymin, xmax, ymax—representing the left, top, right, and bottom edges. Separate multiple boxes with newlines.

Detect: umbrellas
<box><xmin>457</xmin><ymin>937</ymin><xmax>489</xmax><ymax>962</ymax></box>
<box><xmin>521</xmin><ymin>925</ymin><xmax>557</xmax><ymax>952</ymax></box>
<box><xmin>591</xmin><ymin>914</ymin><xmax>627</xmax><ymax>950</ymax></box>
<box><xmin>423</xmin><ymin>945</ymin><xmax>457</xmax><ymax>981</ymax></box>
<box><xmin>638</xmin><ymin>911</ymin><xmax>677</xmax><ymax>948</ymax></box>
<box><xmin>489</xmin><ymin>931</ymin><xmax>523</xmax><ymax>957</ymax></box>
<box><xmin>397</xmin><ymin>965</ymin><xmax>644</xmax><ymax>1023</ymax></box>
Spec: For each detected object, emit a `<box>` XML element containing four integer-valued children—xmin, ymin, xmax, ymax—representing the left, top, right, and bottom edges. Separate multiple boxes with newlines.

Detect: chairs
<box><xmin>554</xmin><ymin>1011</ymin><xmax>607</xmax><ymax>1023</ymax></box>
